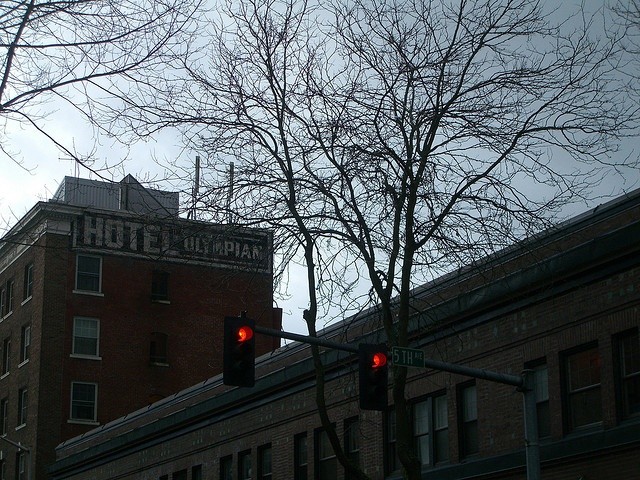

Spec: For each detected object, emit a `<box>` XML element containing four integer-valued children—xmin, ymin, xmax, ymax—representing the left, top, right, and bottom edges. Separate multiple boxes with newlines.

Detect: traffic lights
<box><xmin>223</xmin><ymin>316</ymin><xmax>255</xmax><ymax>387</ymax></box>
<box><xmin>358</xmin><ymin>342</ymin><xmax>388</xmax><ymax>410</ymax></box>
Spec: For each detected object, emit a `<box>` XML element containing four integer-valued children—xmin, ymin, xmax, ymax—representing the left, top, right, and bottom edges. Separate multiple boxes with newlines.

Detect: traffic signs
<box><xmin>391</xmin><ymin>345</ymin><xmax>425</xmax><ymax>368</ymax></box>
